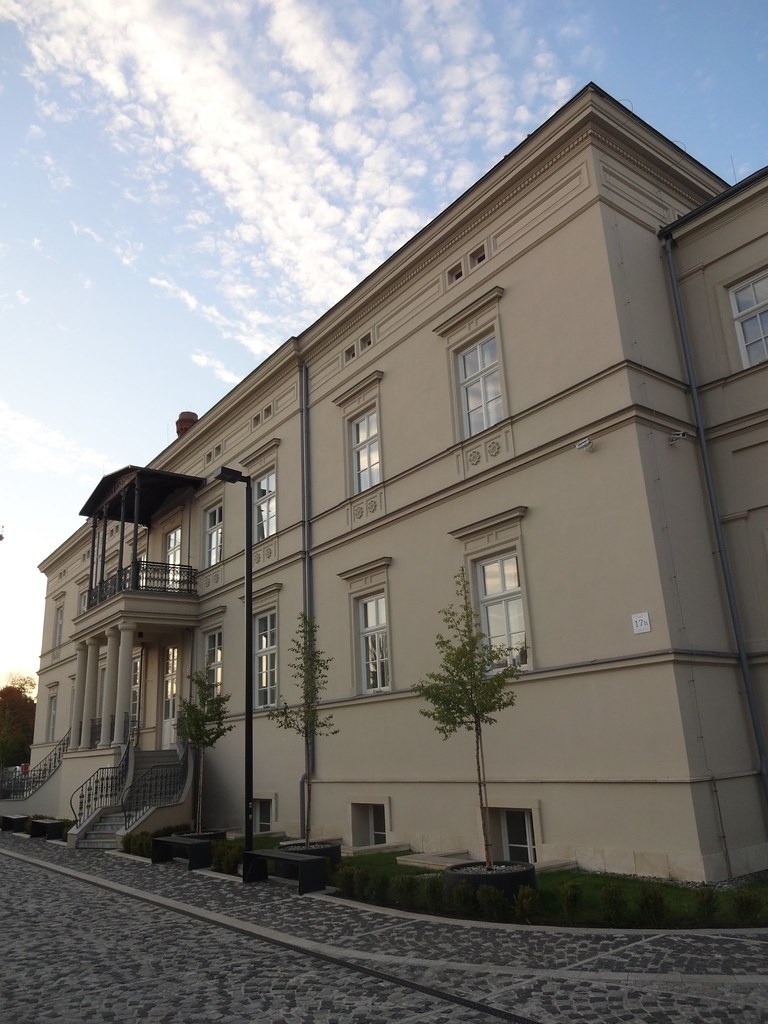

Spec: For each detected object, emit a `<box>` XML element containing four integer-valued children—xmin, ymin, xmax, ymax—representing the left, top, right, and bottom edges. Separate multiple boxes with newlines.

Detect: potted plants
<box><xmin>264</xmin><ymin>612</ymin><xmax>341</xmax><ymax>866</ymax></box>
<box><xmin>171</xmin><ymin>669</ymin><xmax>236</xmax><ymax>840</ymax></box>
<box><xmin>410</xmin><ymin>562</ymin><xmax>536</xmax><ymax>922</ymax></box>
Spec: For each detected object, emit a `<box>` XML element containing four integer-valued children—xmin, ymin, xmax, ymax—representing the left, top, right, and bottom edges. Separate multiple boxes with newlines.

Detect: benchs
<box><xmin>1</xmin><ymin>815</ymin><xmax>29</xmax><ymax>832</ymax></box>
<box><xmin>30</xmin><ymin>819</ymin><xmax>65</xmax><ymax>839</ymax></box>
<box><xmin>241</xmin><ymin>848</ymin><xmax>327</xmax><ymax>895</ymax></box>
<box><xmin>153</xmin><ymin>837</ymin><xmax>207</xmax><ymax>872</ymax></box>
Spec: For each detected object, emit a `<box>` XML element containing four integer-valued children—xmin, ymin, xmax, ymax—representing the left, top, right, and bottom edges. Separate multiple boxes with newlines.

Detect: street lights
<box><xmin>214</xmin><ymin>464</ymin><xmax>254</xmax><ymax>853</ymax></box>
